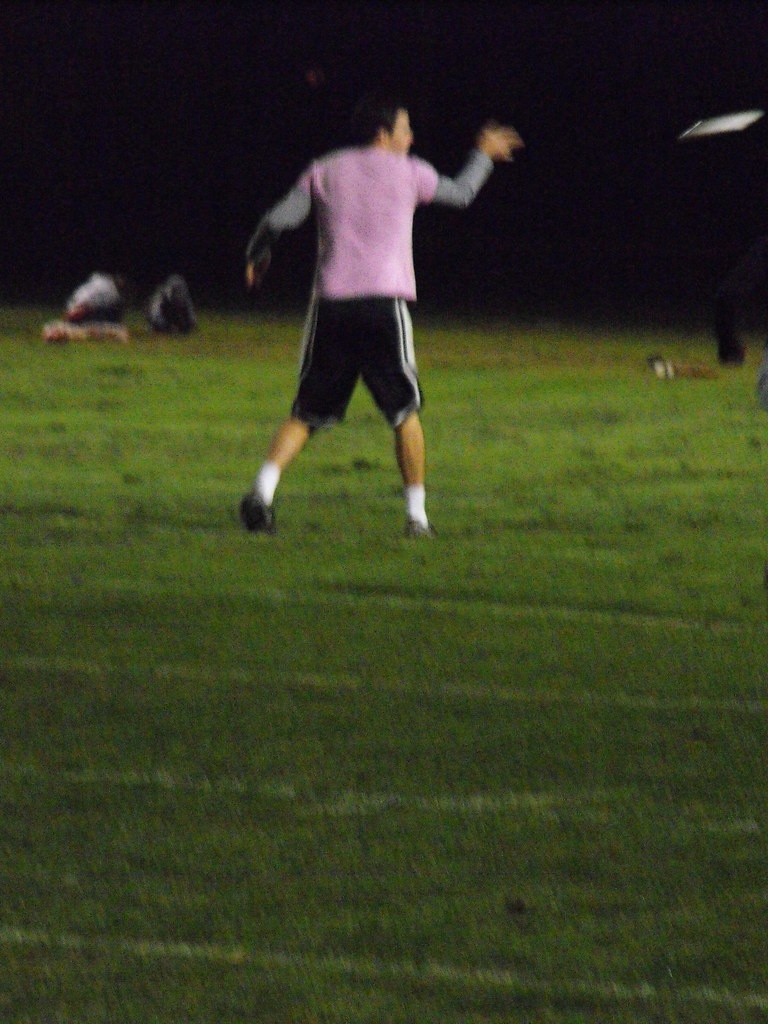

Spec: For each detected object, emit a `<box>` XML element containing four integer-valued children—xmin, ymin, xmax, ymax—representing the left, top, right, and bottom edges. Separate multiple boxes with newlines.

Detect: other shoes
<box><xmin>241</xmin><ymin>491</ymin><xmax>274</xmax><ymax>535</ymax></box>
<box><xmin>407</xmin><ymin>520</ymin><xmax>435</xmax><ymax>540</ymax></box>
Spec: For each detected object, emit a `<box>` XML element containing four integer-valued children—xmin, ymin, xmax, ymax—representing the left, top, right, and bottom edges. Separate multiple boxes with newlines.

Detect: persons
<box><xmin>146</xmin><ymin>275</ymin><xmax>200</xmax><ymax>336</ymax></box>
<box><xmin>240</xmin><ymin>101</ymin><xmax>526</xmax><ymax>538</ymax></box>
<box><xmin>68</xmin><ymin>272</ymin><xmax>123</xmax><ymax>326</ymax></box>
